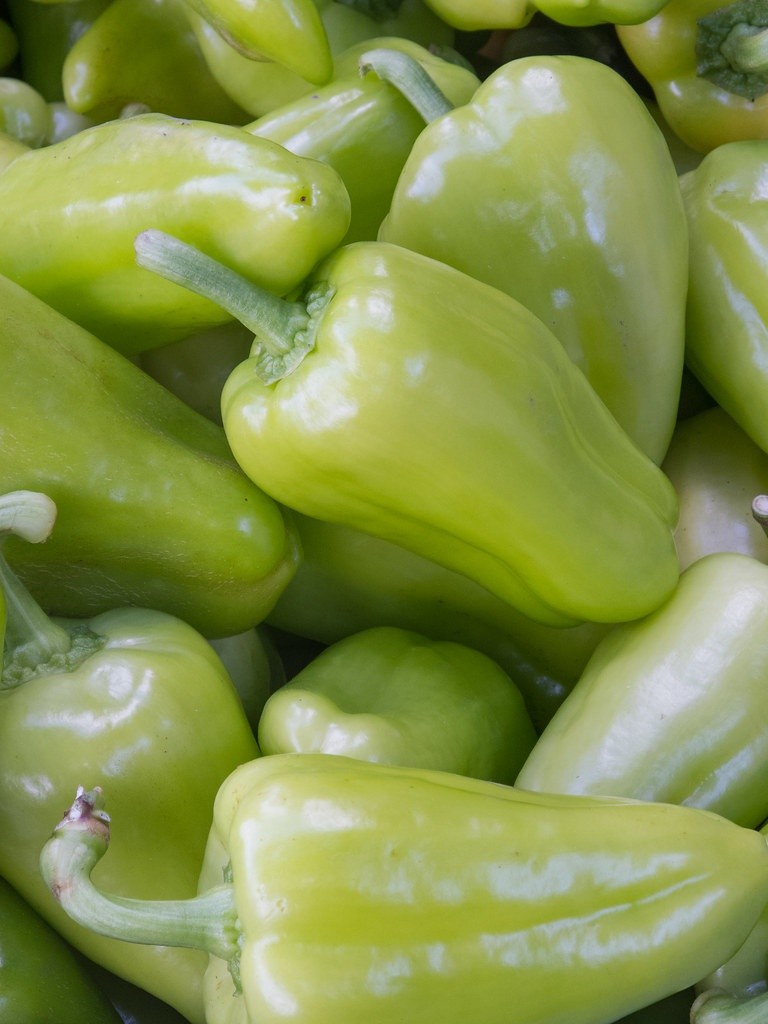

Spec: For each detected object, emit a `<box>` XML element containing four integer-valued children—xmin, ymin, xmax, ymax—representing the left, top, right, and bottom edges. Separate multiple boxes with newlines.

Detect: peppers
<box><xmin>0</xmin><ymin>0</ymin><xmax>768</xmax><ymax>1024</ymax></box>
